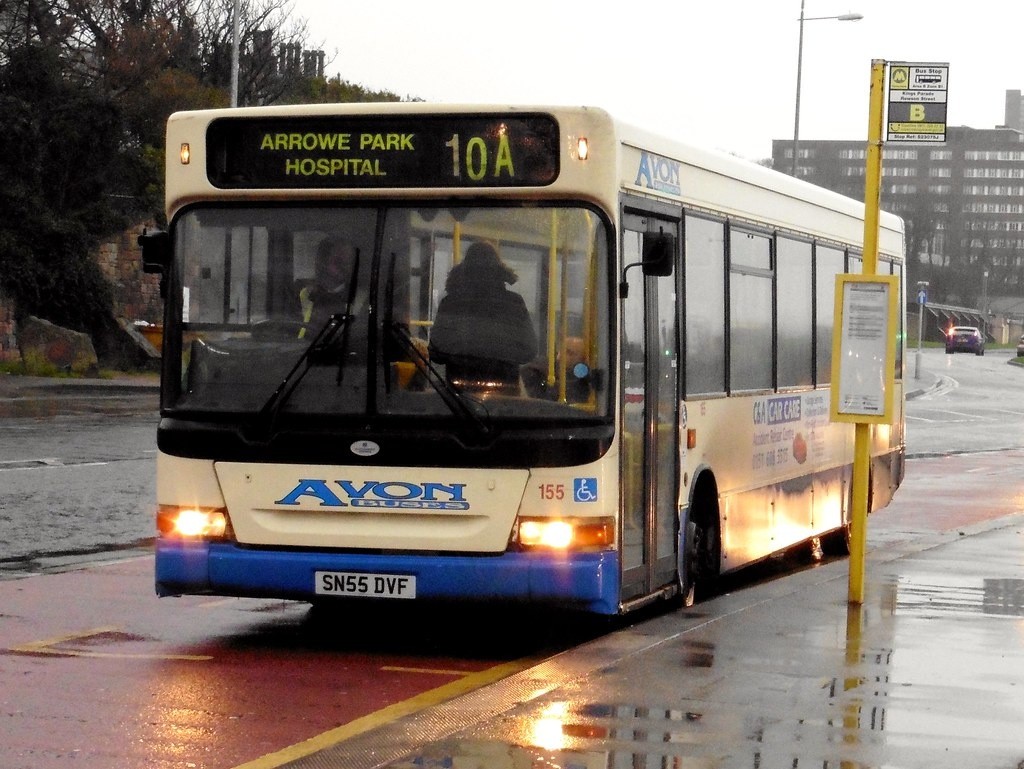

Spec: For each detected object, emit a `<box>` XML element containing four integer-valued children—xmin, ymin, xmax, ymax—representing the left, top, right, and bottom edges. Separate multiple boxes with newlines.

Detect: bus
<box><xmin>137</xmin><ymin>99</ymin><xmax>912</xmax><ymax>613</ymax></box>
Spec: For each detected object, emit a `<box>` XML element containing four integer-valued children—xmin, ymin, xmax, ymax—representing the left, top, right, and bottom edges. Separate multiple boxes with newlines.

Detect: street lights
<box><xmin>794</xmin><ymin>14</ymin><xmax>867</xmax><ymax>179</ymax></box>
<box><xmin>915</xmin><ymin>281</ymin><xmax>930</xmax><ymax>381</ymax></box>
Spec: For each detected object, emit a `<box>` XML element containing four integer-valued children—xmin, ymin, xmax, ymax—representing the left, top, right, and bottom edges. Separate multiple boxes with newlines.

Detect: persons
<box><xmin>427</xmin><ymin>241</ymin><xmax>537</xmax><ymax>397</ymax></box>
<box><xmin>251</xmin><ymin>238</ymin><xmax>385</xmax><ymax>348</ymax></box>
<box><xmin>521</xmin><ymin>338</ymin><xmax>591</xmax><ymax>405</ymax></box>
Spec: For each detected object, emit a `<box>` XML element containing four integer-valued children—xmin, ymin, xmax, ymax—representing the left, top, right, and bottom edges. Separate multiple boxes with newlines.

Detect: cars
<box><xmin>1016</xmin><ymin>334</ymin><xmax>1024</xmax><ymax>358</ymax></box>
<box><xmin>946</xmin><ymin>326</ymin><xmax>986</xmax><ymax>356</ymax></box>
